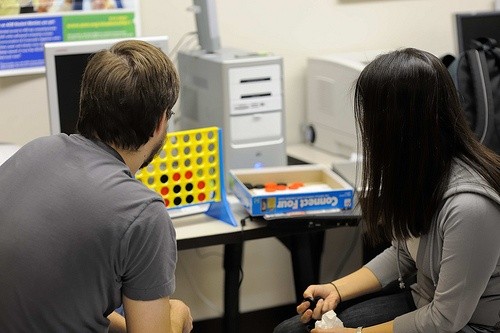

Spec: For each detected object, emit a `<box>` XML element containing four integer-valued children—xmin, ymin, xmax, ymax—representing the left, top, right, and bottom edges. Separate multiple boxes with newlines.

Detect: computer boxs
<box><xmin>176</xmin><ymin>46</ymin><xmax>287</xmax><ymax>195</ymax></box>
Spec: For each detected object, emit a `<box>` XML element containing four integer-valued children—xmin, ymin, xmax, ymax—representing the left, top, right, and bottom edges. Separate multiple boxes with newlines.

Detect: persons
<box><xmin>274</xmin><ymin>47</ymin><xmax>500</xmax><ymax>333</ymax></box>
<box><xmin>0</xmin><ymin>39</ymin><xmax>193</xmax><ymax>333</ymax></box>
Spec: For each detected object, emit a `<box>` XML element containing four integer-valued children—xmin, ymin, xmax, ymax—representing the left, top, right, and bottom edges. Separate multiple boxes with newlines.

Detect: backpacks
<box><xmin>443</xmin><ymin>36</ymin><xmax>500</xmax><ymax>156</ymax></box>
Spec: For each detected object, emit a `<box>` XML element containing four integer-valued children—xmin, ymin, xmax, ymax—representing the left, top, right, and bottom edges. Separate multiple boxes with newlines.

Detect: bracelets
<box><xmin>356</xmin><ymin>325</ymin><xmax>364</xmax><ymax>333</ymax></box>
<box><xmin>327</xmin><ymin>279</ymin><xmax>343</xmax><ymax>304</ymax></box>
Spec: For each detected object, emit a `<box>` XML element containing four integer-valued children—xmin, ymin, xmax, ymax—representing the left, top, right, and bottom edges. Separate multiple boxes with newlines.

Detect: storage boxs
<box><xmin>229</xmin><ymin>166</ymin><xmax>354</xmax><ymax>217</ymax></box>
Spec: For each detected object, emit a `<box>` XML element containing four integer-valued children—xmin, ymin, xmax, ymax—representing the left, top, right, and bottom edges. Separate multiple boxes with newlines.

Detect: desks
<box><xmin>170</xmin><ymin>195</ymin><xmax>362</xmax><ymax>316</ymax></box>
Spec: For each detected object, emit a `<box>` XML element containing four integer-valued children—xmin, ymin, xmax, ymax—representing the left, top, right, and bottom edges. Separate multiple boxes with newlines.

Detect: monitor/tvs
<box><xmin>456</xmin><ymin>10</ymin><xmax>500</xmax><ymax>58</ymax></box>
<box><xmin>43</xmin><ymin>36</ymin><xmax>168</xmax><ymax>136</ymax></box>
